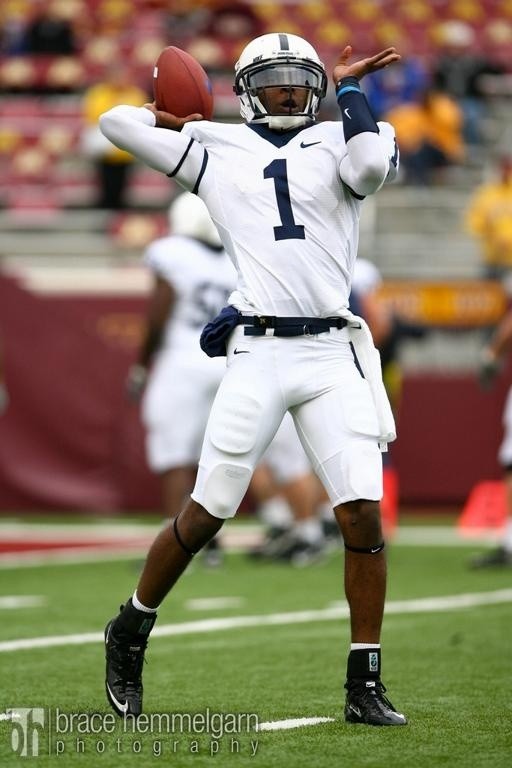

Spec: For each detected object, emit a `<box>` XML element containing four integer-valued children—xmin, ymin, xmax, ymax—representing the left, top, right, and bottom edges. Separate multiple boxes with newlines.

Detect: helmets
<box><xmin>232</xmin><ymin>30</ymin><xmax>329</xmax><ymax>125</ymax></box>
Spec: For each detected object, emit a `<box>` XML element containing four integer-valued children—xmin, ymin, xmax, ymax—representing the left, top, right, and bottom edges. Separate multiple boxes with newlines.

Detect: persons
<box><xmin>0</xmin><ymin>0</ymin><xmax>512</xmax><ymax>569</ymax></box>
<box><xmin>99</xmin><ymin>32</ymin><xmax>408</xmax><ymax>726</ymax></box>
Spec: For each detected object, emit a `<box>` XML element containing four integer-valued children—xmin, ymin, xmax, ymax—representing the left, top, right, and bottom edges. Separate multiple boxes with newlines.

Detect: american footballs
<box><xmin>153</xmin><ymin>46</ymin><xmax>214</xmax><ymax>131</ymax></box>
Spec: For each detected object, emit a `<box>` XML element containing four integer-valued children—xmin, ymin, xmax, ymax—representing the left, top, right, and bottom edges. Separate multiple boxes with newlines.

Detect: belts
<box><xmin>243</xmin><ymin>325</ymin><xmax>331</xmax><ymax>337</ymax></box>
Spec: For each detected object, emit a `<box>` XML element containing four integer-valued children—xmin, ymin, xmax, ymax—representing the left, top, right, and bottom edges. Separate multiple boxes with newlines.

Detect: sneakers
<box><xmin>103</xmin><ymin>617</ymin><xmax>155</xmax><ymax>719</ymax></box>
<box><xmin>202</xmin><ymin>524</ymin><xmax>339</xmax><ymax>566</ymax></box>
<box><xmin>340</xmin><ymin>673</ymin><xmax>408</xmax><ymax>728</ymax></box>
